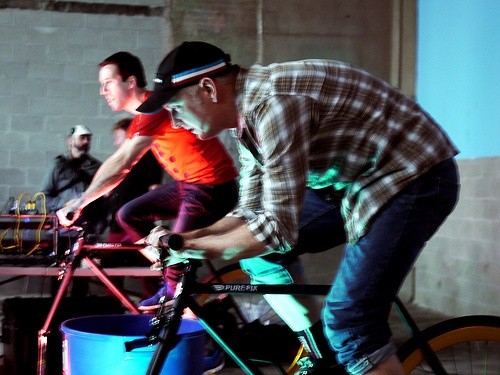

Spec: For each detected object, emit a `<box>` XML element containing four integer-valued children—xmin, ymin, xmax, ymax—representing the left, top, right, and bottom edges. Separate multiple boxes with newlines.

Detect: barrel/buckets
<box><xmin>59</xmin><ymin>314</ymin><xmax>207</xmax><ymax>375</ymax></box>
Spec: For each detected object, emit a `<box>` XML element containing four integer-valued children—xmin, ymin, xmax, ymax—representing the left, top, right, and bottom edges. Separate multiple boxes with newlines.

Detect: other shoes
<box><xmin>201</xmin><ymin>351</ymin><xmax>226</xmax><ymax>375</ymax></box>
<box><xmin>137</xmin><ymin>281</ymin><xmax>175</xmax><ymax>310</ymax></box>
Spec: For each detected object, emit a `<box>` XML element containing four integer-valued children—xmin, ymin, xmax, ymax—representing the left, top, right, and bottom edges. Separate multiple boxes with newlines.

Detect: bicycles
<box><xmin>36</xmin><ymin>212</ymin><xmax>293</xmax><ymax>375</ymax></box>
<box><xmin>125</xmin><ymin>225</ymin><xmax>500</xmax><ymax>375</ymax></box>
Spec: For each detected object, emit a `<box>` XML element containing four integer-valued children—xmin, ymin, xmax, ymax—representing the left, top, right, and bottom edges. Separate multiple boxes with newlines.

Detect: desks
<box><xmin>0</xmin><ymin>264</ymin><xmax>164</xmax><ymax>299</ymax></box>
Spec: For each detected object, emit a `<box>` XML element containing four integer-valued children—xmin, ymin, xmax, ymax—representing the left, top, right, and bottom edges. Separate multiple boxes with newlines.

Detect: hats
<box><xmin>69</xmin><ymin>125</ymin><xmax>93</xmax><ymax>136</ymax></box>
<box><xmin>135</xmin><ymin>40</ymin><xmax>232</xmax><ymax>114</ymax></box>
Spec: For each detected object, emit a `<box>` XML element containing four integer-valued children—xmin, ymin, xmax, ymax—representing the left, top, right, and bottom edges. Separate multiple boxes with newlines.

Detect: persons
<box><xmin>134</xmin><ymin>41</ymin><xmax>461</xmax><ymax>375</ymax></box>
<box><xmin>55</xmin><ymin>51</ymin><xmax>241</xmax><ymax>310</ymax></box>
<box><xmin>38</xmin><ymin>118</ymin><xmax>161</xmax><ymax>298</ymax></box>
